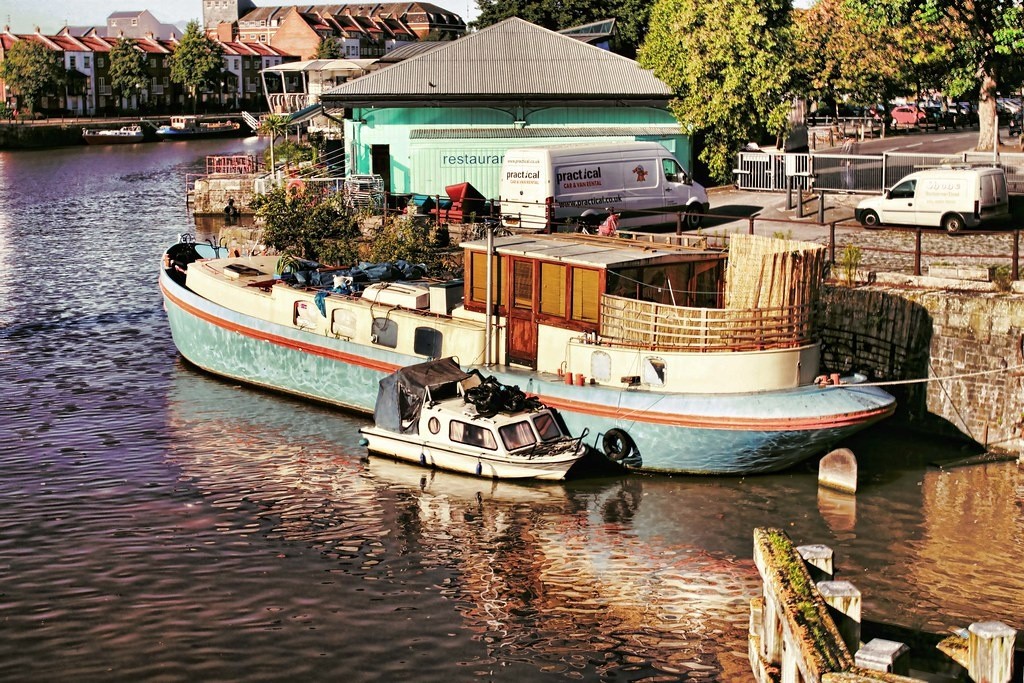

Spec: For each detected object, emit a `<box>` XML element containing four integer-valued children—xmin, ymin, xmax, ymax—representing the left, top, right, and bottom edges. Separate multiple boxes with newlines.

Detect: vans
<box><xmin>499</xmin><ymin>140</ymin><xmax>710</xmax><ymax>236</ymax></box>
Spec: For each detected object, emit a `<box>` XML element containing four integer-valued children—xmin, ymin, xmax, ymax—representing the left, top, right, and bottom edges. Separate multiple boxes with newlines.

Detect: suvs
<box><xmin>855</xmin><ymin>162</ymin><xmax>1010</xmax><ymax>236</ymax></box>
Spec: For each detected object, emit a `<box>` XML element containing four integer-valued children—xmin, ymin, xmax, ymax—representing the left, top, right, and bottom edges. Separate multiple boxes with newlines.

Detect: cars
<box><xmin>1008</xmin><ymin>114</ymin><xmax>1023</xmax><ymax>136</ymax></box>
<box><xmin>890</xmin><ymin>98</ymin><xmax>1024</xmax><ymax>125</ymax></box>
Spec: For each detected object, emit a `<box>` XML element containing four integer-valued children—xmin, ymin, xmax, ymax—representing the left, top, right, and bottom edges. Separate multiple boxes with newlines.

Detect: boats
<box><xmin>155</xmin><ymin>114</ymin><xmax>240</xmax><ymax>140</ymax></box>
<box><xmin>359</xmin><ymin>353</ymin><xmax>588</xmax><ymax>481</ymax></box>
<box><xmin>158</xmin><ymin>212</ymin><xmax>897</xmax><ymax>479</ymax></box>
<box><xmin>82</xmin><ymin>124</ymin><xmax>144</xmax><ymax>144</ymax></box>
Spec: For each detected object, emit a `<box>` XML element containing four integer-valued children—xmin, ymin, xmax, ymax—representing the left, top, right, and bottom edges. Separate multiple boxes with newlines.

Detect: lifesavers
<box><xmin>286</xmin><ymin>181</ymin><xmax>306</xmax><ymax>198</ymax></box>
<box><xmin>603</xmin><ymin>429</ymin><xmax>629</xmax><ymax>458</ymax></box>
